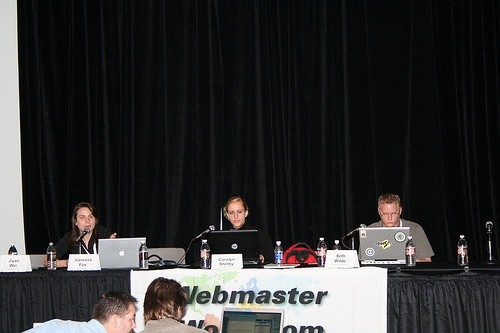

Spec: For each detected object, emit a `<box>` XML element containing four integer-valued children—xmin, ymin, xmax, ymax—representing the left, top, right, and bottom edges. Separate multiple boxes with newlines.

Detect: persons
<box><xmin>139</xmin><ymin>277</ymin><xmax>222</xmax><ymax>333</ymax></box>
<box><xmin>366</xmin><ymin>193</ymin><xmax>434</xmax><ymax>262</ymax></box>
<box><xmin>223</xmin><ymin>196</ymin><xmax>275</xmax><ymax>263</ymax></box>
<box><xmin>45</xmin><ymin>202</ymin><xmax>117</xmax><ymax>268</ymax></box>
<box><xmin>21</xmin><ymin>290</ymin><xmax>139</xmax><ymax>333</ymax></box>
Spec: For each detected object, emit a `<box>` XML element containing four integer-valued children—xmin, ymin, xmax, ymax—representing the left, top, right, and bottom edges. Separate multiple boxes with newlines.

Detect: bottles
<box><xmin>274</xmin><ymin>241</ymin><xmax>284</xmax><ymax>266</ymax></box>
<box><xmin>47</xmin><ymin>242</ymin><xmax>56</xmax><ymax>271</ymax></box>
<box><xmin>317</xmin><ymin>238</ymin><xmax>327</xmax><ymax>267</ymax></box>
<box><xmin>200</xmin><ymin>240</ymin><xmax>211</xmax><ymax>269</ymax></box>
<box><xmin>457</xmin><ymin>235</ymin><xmax>468</xmax><ymax>266</ymax></box>
<box><xmin>8</xmin><ymin>243</ymin><xmax>17</xmax><ymax>255</ymax></box>
<box><xmin>405</xmin><ymin>236</ymin><xmax>416</xmax><ymax>266</ymax></box>
<box><xmin>333</xmin><ymin>240</ymin><xmax>341</xmax><ymax>250</ymax></box>
<box><xmin>139</xmin><ymin>241</ymin><xmax>149</xmax><ymax>270</ymax></box>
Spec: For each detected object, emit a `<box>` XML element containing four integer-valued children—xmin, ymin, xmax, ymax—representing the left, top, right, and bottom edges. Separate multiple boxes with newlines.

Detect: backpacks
<box><xmin>284</xmin><ymin>242</ymin><xmax>318</xmax><ymax>264</ymax></box>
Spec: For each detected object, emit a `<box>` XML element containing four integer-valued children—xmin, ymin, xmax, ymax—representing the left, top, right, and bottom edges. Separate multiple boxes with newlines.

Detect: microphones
<box><xmin>196</xmin><ymin>225</ymin><xmax>215</xmax><ymax>239</ymax></box>
<box><xmin>347</xmin><ymin>224</ymin><xmax>366</xmax><ymax>237</ymax></box>
<box><xmin>485</xmin><ymin>221</ymin><xmax>493</xmax><ymax>233</ymax></box>
<box><xmin>75</xmin><ymin>227</ymin><xmax>90</xmax><ymax>242</ymax></box>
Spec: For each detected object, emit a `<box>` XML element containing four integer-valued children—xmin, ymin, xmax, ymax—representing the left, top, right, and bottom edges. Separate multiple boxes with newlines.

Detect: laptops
<box><xmin>221</xmin><ymin>308</ymin><xmax>284</xmax><ymax>333</ymax></box>
<box><xmin>358</xmin><ymin>227</ymin><xmax>410</xmax><ymax>261</ymax></box>
<box><xmin>210</xmin><ymin>229</ymin><xmax>261</xmax><ymax>265</ymax></box>
<box><xmin>98</xmin><ymin>237</ymin><xmax>147</xmax><ymax>269</ymax></box>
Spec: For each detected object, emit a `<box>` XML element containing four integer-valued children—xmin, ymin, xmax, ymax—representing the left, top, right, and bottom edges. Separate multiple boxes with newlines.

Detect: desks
<box><xmin>0</xmin><ymin>267</ymin><xmax>500</xmax><ymax>333</ymax></box>
